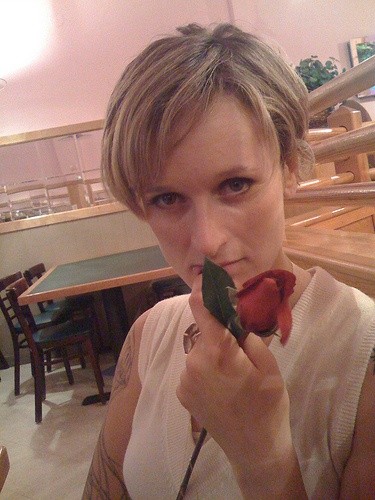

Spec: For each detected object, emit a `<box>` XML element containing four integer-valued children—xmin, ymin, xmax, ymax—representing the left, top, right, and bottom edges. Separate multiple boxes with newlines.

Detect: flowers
<box><xmin>175</xmin><ymin>254</ymin><xmax>297</xmax><ymax>500</ymax></box>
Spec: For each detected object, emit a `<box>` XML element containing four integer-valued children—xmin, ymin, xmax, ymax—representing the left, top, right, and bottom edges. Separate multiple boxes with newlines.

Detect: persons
<box><xmin>80</xmin><ymin>23</ymin><xmax>375</xmax><ymax>500</ymax></box>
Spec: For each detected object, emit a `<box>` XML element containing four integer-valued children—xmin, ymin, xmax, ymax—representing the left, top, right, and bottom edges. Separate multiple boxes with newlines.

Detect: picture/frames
<box><xmin>348</xmin><ymin>36</ymin><xmax>375</xmax><ymax>99</ymax></box>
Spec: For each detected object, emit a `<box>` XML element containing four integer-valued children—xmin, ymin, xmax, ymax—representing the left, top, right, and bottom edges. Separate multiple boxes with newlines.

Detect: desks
<box><xmin>18</xmin><ymin>246</ymin><xmax>177</xmax><ymax>330</ymax></box>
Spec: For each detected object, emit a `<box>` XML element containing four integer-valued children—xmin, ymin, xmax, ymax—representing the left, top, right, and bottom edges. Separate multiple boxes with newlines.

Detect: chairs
<box><xmin>0</xmin><ymin>166</ymin><xmax>119</xmax><ymax>223</ymax></box>
<box><xmin>0</xmin><ymin>262</ymin><xmax>106</xmax><ymax>423</ymax></box>
